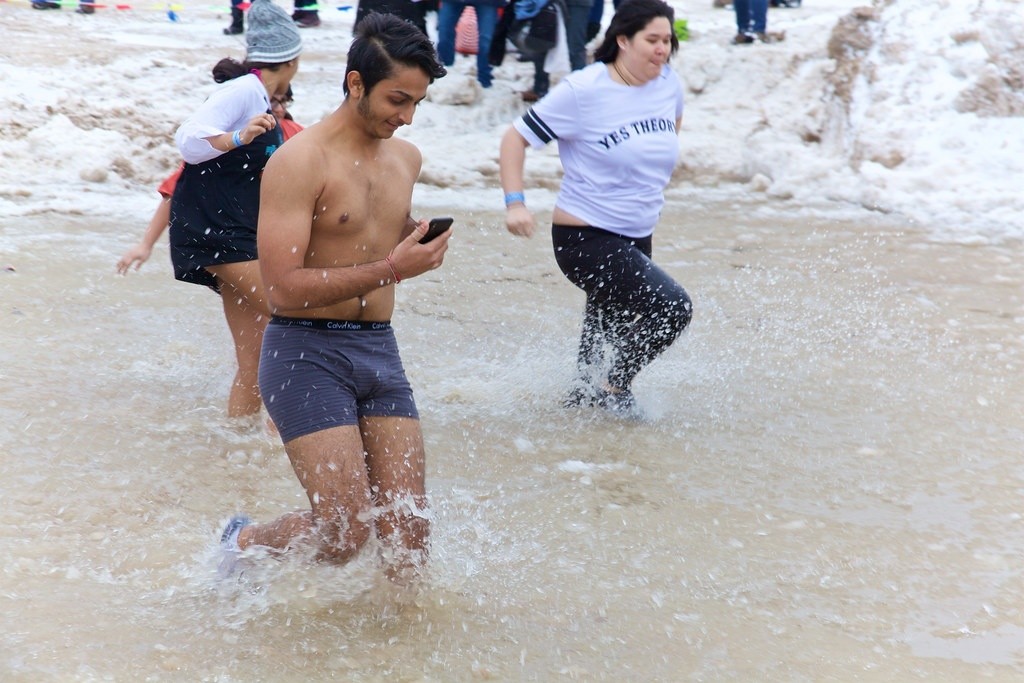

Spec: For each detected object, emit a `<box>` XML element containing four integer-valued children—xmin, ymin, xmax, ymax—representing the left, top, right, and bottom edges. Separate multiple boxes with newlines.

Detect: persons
<box><xmin>268</xmin><ymin>81</ymin><xmax>294</xmax><ymax>120</ymax></box>
<box><xmin>501</xmin><ymin>1</ymin><xmax>694</xmax><ymax>423</ymax></box>
<box><xmin>220</xmin><ymin>13</ymin><xmax>452</xmax><ymax>580</ymax></box>
<box><xmin>31</xmin><ymin>0</ymin><xmax>770</xmax><ymax>103</ymax></box>
<box><xmin>168</xmin><ymin>1</ymin><xmax>306</xmax><ymax>438</ymax></box>
<box><xmin>115</xmin><ymin>58</ymin><xmax>308</xmax><ymax>274</ymax></box>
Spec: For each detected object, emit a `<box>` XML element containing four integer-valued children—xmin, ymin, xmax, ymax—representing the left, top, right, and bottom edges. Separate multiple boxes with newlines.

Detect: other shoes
<box><xmin>562</xmin><ymin>383</ymin><xmax>596</xmax><ymax>410</ymax></box>
<box><xmin>224</xmin><ymin>26</ymin><xmax>242</xmax><ymax>35</ymax></box>
<box><xmin>221</xmin><ymin>517</ymin><xmax>250</xmax><ymax>549</ymax></box>
<box><xmin>594</xmin><ymin>389</ymin><xmax>637</xmax><ymax>417</ymax></box>
<box><xmin>735</xmin><ymin>32</ymin><xmax>765</xmax><ymax>44</ymax></box>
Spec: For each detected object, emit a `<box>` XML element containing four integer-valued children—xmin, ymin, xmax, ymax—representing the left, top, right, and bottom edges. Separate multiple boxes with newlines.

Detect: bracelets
<box><xmin>504</xmin><ymin>192</ymin><xmax>524</xmax><ymax>204</ymax></box>
<box><xmin>386</xmin><ymin>257</ymin><xmax>401</xmax><ymax>285</ymax></box>
<box><xmin>233</xmin><ymin>129</ymin><xmax>244</xmax><ymax>148</ymax></box>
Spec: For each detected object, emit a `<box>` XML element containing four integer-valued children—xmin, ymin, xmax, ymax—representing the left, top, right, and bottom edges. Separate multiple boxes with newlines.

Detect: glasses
<box><xmin>270</xmin><ymin>97</ymin><xmax>294</xmax><ymax>109</ymax></box>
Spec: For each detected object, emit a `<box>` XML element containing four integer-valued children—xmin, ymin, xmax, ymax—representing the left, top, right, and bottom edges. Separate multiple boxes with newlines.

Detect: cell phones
<box><xmin>418</xmin><ymin>218</ymin><xmax>454</xmax><ymax>244</ymax></box>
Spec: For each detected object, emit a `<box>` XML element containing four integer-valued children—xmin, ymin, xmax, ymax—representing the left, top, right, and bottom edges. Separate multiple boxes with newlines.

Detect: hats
<box><xmin>244</xmin><ymin>1</ymin><xmax>304</xmax><ymax>64</ymax></box>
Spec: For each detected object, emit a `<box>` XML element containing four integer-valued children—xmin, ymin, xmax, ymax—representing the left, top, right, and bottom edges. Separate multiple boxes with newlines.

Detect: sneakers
<box><xmin>522</xmin><ymin>90</ymin><xmax>541</xmax><ymax>101</ymax></box>
